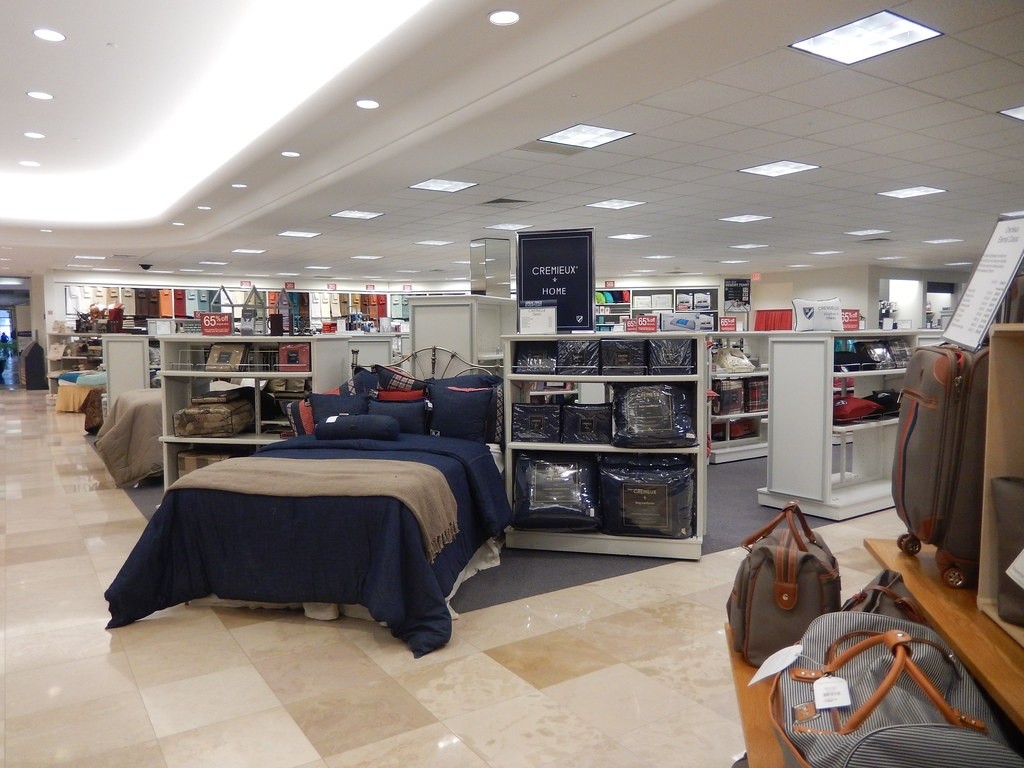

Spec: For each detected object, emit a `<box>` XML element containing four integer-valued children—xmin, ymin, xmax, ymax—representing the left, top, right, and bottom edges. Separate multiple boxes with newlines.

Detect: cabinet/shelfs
<box><xmin>155</xmin><ymin>335</ymin><xmax>354</xmax><ymax>505</ymax></box>
<box><xmin>46</xmin><ymin>331</ymin><xmax>107</xmax><ymax>397</ymax></box>
<box><xmin>709</xmin><ymin>330</ymin><xmax>785</xmax><ymax>464</ymax></box>
<box><xmin>755</xmin><ymin>329</ymin><xmax>942</xmax><ymax>520</ymax></box>
<box><xmin>723</xmin><ymin>536</ymin><xmax>1024</xmax><ymax>768</ymax></box>
<box><xmin>595</xmin><ymin>285</ymin><xmax>721</xmax><ymax>333</ymax></box>
<box><xmin>106</xmin><ymin>334</ymin><xmax>160</xmax><ymax>415</ymax></box>
<box><xmin>499</xmin><ymin>331</ymin><xmax>712</xmax><ymax>563</ymax></box>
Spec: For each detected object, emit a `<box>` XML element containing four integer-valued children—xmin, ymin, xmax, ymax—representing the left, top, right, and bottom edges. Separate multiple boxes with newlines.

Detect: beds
<box><xmin>55</xmin><ymin>366</ymin><xmax>107</xmax><ymax>412</ymax></box>
<box><xmin>105</xmin><ymin>345</ymin><xmax>506</xmax><ymax>658</ymax></box>
<box><xmin>93</xmin><ymin>375</ymin><xmax>267</xmax><ymax>488</ymax></box>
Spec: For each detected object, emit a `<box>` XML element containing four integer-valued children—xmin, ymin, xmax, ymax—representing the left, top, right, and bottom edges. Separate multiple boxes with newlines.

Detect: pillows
<box><xmin>791</xmin><ymin>297</ymin><xmax>845</xmax><ymax>333</ymax></box>
<box><xmin>288</xmin><ymin>365</ymin><xmax>502</xmax><ymax>442</ymax></box>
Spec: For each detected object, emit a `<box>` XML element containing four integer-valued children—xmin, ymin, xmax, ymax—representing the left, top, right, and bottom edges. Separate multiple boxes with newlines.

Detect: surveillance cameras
<box><xmin>139</xmin><ymin>256</ymin><xmax>152</xmax><ymax>270</ymax></box>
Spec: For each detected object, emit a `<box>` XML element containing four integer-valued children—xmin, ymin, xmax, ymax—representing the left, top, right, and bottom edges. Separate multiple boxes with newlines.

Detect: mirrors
<box><xmin>470</xmin><ymin>238</ymin><xmax>511</xmax><ymax>298</ymax></box>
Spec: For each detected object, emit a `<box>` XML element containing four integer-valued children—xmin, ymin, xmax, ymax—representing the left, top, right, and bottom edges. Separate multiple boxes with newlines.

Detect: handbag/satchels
<box><xmin>725</xmin><ymin>501</ymin><xmax>856</xmax><ymax>647</ymax></box>
<box><xmin>840</xmin><ymin>572</ymin><xmax>926</xmax><ymax>620</ymax></box>
<box><xmin>772</xmin><ymin>612</ymin><xmax>1022</xmax><ymax>768</ymax></box>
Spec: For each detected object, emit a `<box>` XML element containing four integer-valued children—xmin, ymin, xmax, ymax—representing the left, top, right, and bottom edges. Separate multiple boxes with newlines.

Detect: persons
<box><xmin>1</xmin><ymin>332</ymin><xmax>9</xmax><ymax>343</ymax></box>
<box><xmin>10</xmin><ymin>328</ymin><xmax>16</xmax><ymax>339</ymax></box>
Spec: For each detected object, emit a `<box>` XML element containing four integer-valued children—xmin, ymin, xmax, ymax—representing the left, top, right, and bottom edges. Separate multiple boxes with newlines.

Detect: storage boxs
<box><xmin>594</xmin><ymin>291</ymin><xmax>715</xmax><ymax>331</ymax></box>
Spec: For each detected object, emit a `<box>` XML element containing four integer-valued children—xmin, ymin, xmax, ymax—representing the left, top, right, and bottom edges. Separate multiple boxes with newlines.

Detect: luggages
<box><xmin>897</xmin><ymin>343</ymin><xmax>994</xmax><ymax>587</ymax></box>
